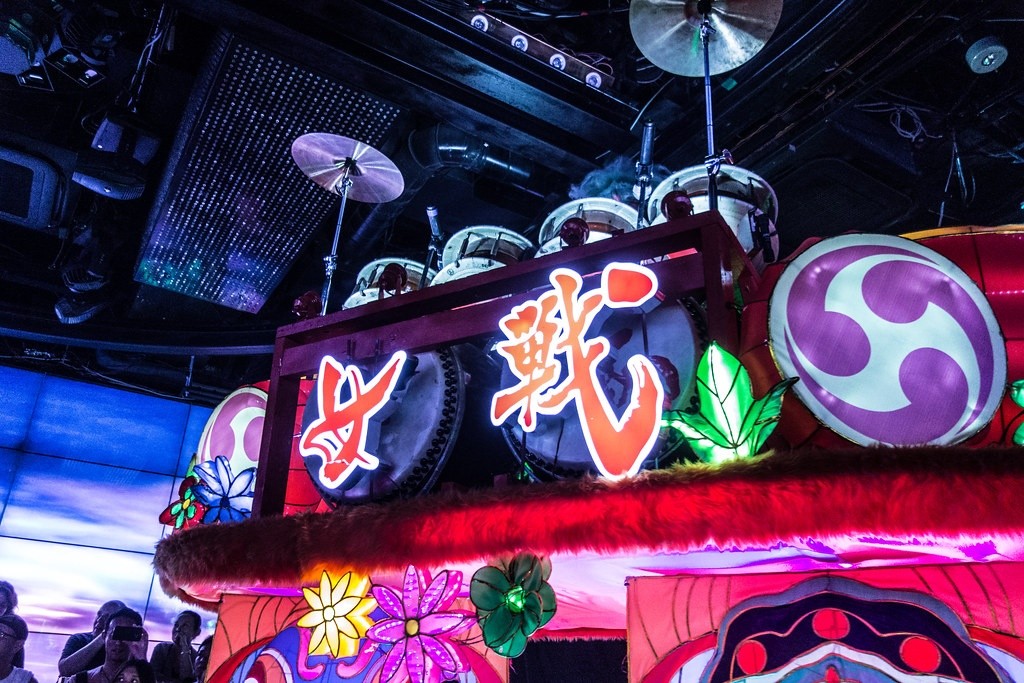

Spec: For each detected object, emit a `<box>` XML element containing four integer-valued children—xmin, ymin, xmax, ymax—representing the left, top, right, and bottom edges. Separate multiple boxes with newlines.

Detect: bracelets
<box><xmin>180</xmin><ymin>650</ymin><xmax>192</xmax><ymax>655</ymax></box>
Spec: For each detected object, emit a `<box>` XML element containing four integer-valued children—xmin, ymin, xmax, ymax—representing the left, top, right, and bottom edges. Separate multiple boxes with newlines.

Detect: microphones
<box><xmin>427</xmin><ymin>205</ymin><xmax>444</xmax><ymax>271</ymax></box>
<box><xmin>637</xmin><ymin>119</ymin><xmax>656</xmax><ymax>182</ymax></box>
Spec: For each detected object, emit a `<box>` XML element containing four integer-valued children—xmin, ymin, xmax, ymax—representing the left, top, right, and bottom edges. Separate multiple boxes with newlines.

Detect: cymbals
<box><xmin>629</xmin><ymin>0</ymin><xmax>785</xmax><ymax>79</ymax></box>
<box><xmin>290</xmin><ymin>131</ymin><xmax>406</xmax><ymax>205</ymax></box>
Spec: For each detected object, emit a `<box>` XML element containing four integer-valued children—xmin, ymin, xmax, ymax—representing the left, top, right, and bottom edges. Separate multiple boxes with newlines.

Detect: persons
<box><xmin>58</xmin><ymin>600</ymin><xmax>128</xmax><ymax>676</ymax></box>
<box><xmin>0</xmin><ymin>580</ymin><xmax>24</xmax><ymax>668</ymax></box>
<box><xmin>114</xmin><ymin>658</ymin><xmax>155</xmax><ymax>683</ymax></box>
<box><xmin>150</xmin><ymin>610</ymin><xmax>202</xmax><ymax>683</ymax></box>
<box><xmin>194</xmin><ymin>634</ymin><xmax>214</xmax><ymax>683</ymax></box>
<box><xmin>0</xmin><ymin>614</ymin><xmax>38</xmax><ymax>683</ymax></box>
<box><xmin>57</xmin><ymin>607</ymin><xmax>155</xmax><ymax>683</ymax></box>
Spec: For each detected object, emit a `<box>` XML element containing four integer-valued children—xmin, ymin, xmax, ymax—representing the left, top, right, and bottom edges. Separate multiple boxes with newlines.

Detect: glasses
<box><xmin>0</xmin><ymin>631</ymin><xmax>19</xmax><ymax>640</ymax></box>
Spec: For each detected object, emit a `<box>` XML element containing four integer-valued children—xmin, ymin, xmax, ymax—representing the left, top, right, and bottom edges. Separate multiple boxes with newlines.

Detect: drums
<box><xmin>654</xmin><ymin>162</ymin><xmax>781</xmax><ymax>276</ymax></box>
<box><xmin>342</xmin><ymin>257</ymin><xmax>439</xmax><ymax>311</ymax></box>
<box><xmin>533</xmin><ymin>195</ymin><xmax>650</xmax><ymax>259</ymax></box>
<box><xmin>429</xmin><ymin>224</ymin><xmax>537</xmax><ymax>287</ymax></box>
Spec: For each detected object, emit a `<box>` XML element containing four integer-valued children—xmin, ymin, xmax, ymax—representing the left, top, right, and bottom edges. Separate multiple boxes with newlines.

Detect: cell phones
<box><xmin>112</xmin><ymin>626</ymin><xmax>143</xmax><ymax>641</ymax></box>
